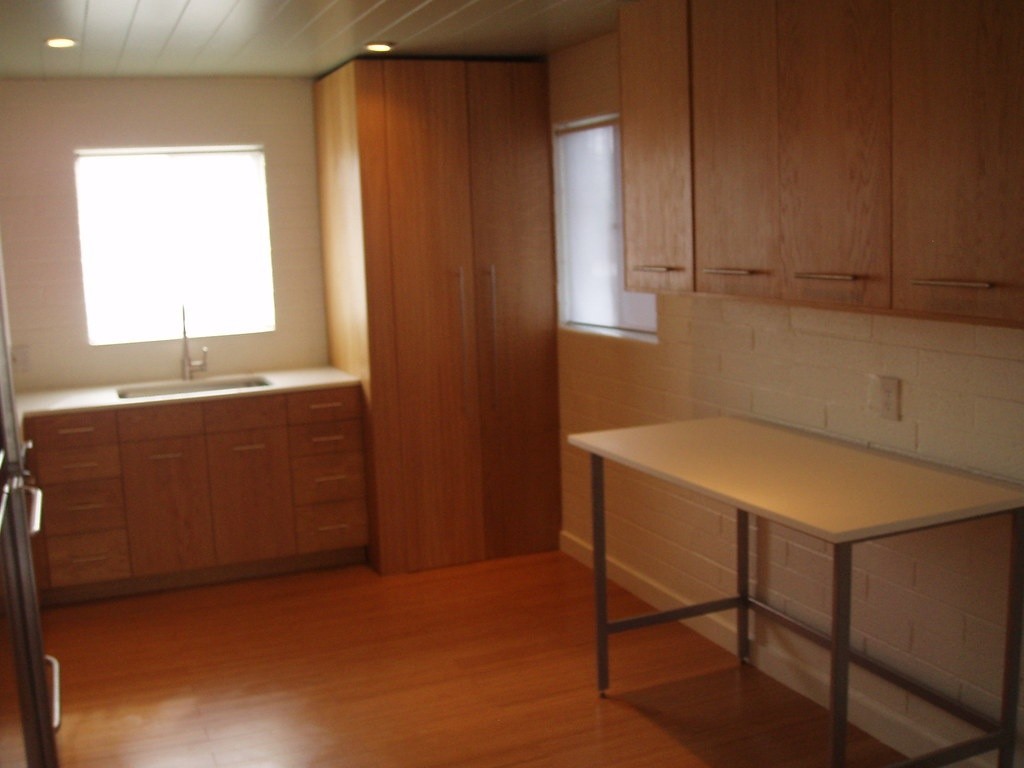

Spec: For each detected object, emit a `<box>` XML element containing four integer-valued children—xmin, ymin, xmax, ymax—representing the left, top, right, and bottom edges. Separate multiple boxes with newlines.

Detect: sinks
<box><xmin>116</xmin><ymin>375</ymin><xmax>273</xmax><ymax>400</ymax></box>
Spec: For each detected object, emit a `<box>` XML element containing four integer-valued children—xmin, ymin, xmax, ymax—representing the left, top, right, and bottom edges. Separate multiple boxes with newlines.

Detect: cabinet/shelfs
<box><xmin>313</xmin><ymin>58</ymin><xmax>562</xmax><ymax>576</ymax></box>
<box><xmin>21</xmin><ymin>387</ymin><xmax>370</xmax><ymax>605</ymax></box>
<box><xmin>618</xmin><ymin>0</ymin><xmax>1024</xmax><ymax>329</ymax></box>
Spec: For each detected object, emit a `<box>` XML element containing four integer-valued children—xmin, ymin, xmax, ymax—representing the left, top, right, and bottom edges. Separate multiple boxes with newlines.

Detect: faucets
<box><xmin>179</xmin><ymin>304</ymin><xmax>208</xmax><ymax>381</ymax></box>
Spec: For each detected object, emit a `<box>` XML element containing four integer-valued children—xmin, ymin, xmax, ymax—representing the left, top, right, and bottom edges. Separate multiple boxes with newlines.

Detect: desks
<box><xmin>568</xmin><ymin>416</ymin><xmax>1024</xmax><ymax>768</ymax></box>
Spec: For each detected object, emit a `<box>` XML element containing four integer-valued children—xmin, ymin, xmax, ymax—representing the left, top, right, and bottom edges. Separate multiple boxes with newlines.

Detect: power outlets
<box><xmin>882</xmin><ymin>379</ymin><xmax>901</xmax><ymax>421</ymax></box>
